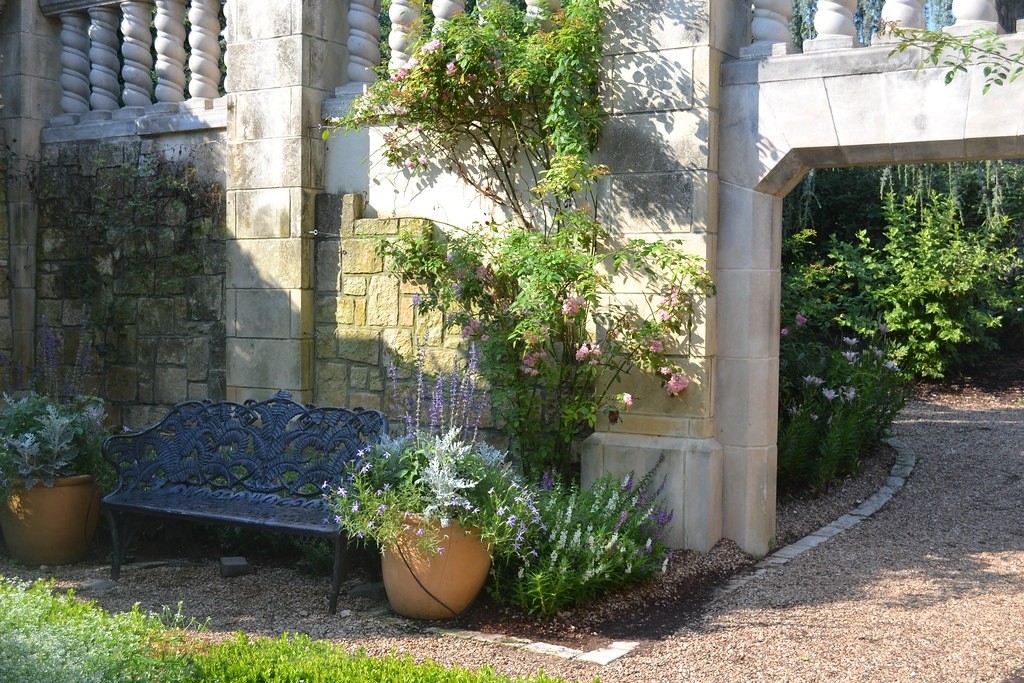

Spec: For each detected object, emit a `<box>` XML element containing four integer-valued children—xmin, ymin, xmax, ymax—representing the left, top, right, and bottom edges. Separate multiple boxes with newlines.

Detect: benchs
<box><xmin>101</xmin><ymin>389</ymin><xmax>388</xmax><ymax>615</ymax></box>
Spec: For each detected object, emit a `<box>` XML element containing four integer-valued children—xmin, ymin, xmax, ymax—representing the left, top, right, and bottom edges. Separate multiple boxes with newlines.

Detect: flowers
<box><xmin>322</xmin><ymin>338</ymin><xmax>548</xmax><ymax>560</ymax></box>
<box><xmin>0</xmin><ymin>317</ymin><xmax>109</xmax><ymax>497</ymax></box>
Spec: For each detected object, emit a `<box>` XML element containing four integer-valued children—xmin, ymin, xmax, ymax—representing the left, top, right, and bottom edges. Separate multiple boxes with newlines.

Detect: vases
<box><xmin>379</xmin><ymin>512</ymin><xmax>495</xmax><ymax>620</ymax></box>
<box><xmin>0</xmin><ymin>474</ymin><xmax>100</xmax><ymax>566</ymax></box>
